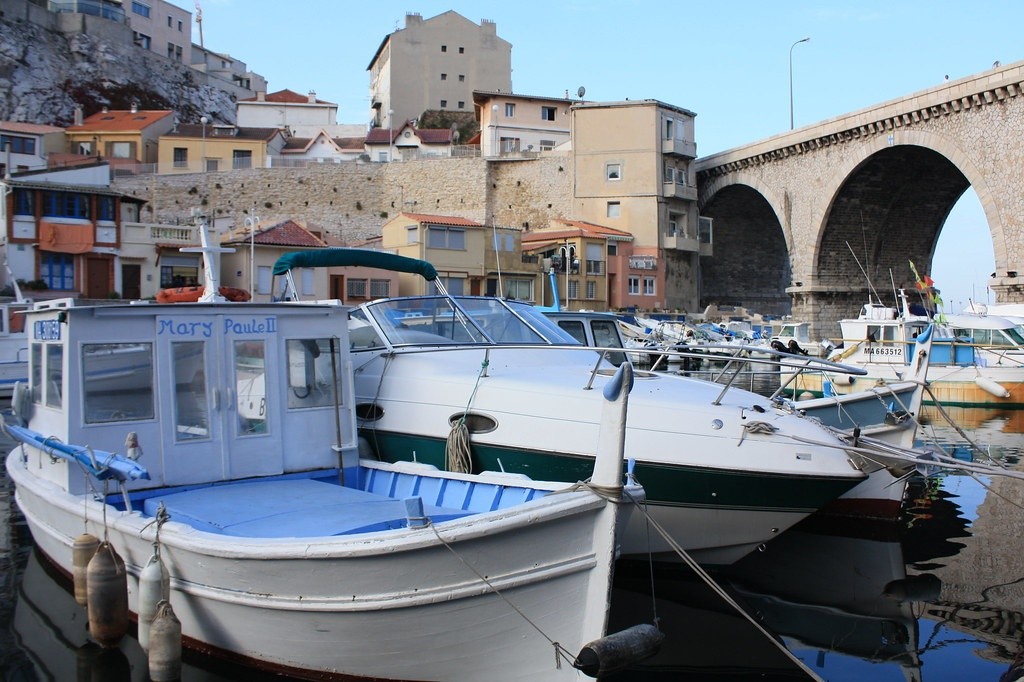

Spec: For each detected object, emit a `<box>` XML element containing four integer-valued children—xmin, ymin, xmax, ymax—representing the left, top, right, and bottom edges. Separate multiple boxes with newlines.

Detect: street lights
<box><xmin>789</xmin><ymin>37</ymin><xmax>811</xmax><ymax>132</ymax></box>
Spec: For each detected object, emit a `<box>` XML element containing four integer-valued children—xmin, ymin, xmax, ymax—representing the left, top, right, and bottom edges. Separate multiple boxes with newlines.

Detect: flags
<box><xmin>908</xmin><ymin>259</ymin><xmax>947</xmax><ymax>323</ymax></box>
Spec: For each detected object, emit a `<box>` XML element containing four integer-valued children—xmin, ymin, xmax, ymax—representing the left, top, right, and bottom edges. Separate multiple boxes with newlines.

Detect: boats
<box><xmin>5</xmin><ymin>295</ymin><xmax>650</xmax><ymax>682</ymax></box>
<box><xmin>357</xmin><ymin>283</ymin><xmax>1023</xmax><ymax>462</ymax></box>
<box><xmin>235</xmin><ymin>246</ymin><xmax>871</xmax><ymax>587</ymax></box>
<box><xmin>0</xmin><ymin>323</ymin><xmax>250</xmax><ymax>399</ymax></box>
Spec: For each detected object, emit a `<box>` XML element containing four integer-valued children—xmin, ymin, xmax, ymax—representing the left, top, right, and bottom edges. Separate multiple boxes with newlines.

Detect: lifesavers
<box><xmin>157</xmin><ymin>287</ymin><xmax>252</xmax><ymax>302</ymax></box>
